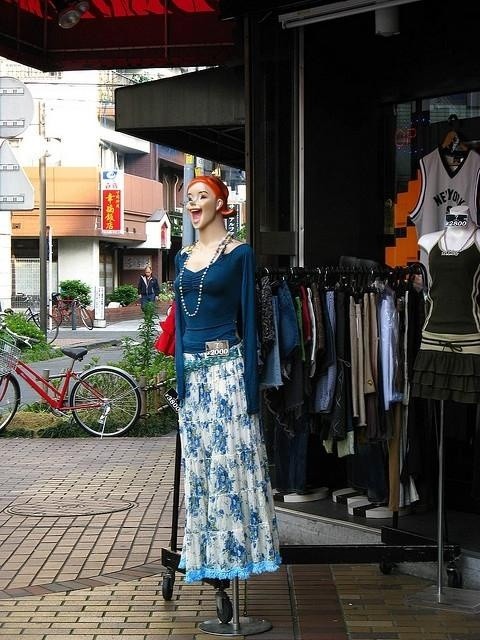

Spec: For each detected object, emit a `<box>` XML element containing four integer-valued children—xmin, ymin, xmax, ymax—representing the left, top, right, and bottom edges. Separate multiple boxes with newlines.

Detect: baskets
<box><xmin>0</xmin><ymin>338</ymin><xmax>21</xmax><ymax>377</ymax></box>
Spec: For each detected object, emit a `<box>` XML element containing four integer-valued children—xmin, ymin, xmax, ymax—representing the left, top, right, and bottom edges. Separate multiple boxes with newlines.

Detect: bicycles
<box><xmin>0</xmin><ymin>291</ymin><xmax>60</xmax><ymax>346</ymax></box>
<box><xmin>0</xmin><ymin>322</ymin><xmax>143</xmax><ymax>439</ymax></box>
<box><xmin>39</xmin><ymin>291</ymin><xmax>95</xmax><ymax>331</ymax></box>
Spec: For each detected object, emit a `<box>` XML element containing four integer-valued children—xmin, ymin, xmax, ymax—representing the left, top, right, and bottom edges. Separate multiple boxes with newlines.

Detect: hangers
<box><xmin>255</xmin><ymin>264</ymin><xmax>418</xmax><ymax>288</ymax></box>
<box><xmin>441</xmin><ymin>114</ymin><xmax>469</xmax><ymax>158</ymax></box>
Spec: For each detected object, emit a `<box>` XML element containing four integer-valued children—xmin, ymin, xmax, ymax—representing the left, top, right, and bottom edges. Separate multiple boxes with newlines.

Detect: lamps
<box><xmin>57</xmin><ymin>0</ymin><xmax>89</xmax><ymax>30</ymax></box>
<box><xmin>59</xmin><ymin>280</ymin><xmax>92</xmax><ymax>308</ymax></box>
<box><xmin>374</xmin><ymin>0</ymin><xmax>402</xmax><ymax>36</ymax></box>
<box><xmin>279</xmin><ymin>1</ymin><xmax>417</xmax><ymax>29</ymax></box>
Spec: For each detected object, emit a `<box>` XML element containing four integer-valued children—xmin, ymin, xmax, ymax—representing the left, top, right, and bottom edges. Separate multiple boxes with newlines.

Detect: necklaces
<box><xmin>178</xmin><ymin>232</ymin><xmax>233</xmax><ymax>318</ymax></box>
<box><xmin>440</xmin><ymin>227</ymin><xmax>477</xmax><ymax>258</ymax></box>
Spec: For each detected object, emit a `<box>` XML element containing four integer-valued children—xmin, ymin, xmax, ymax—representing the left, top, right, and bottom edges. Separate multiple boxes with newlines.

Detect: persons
<box><xmin>137</xmin><ymin>266</ymin><xmax>161</xmax><ymax>318</ymax></box>
<box><xmin>154</xmin><ymin>173</ymin><xmax>288</xmax><ymax>586</ymax></box>
<box><xmin>404</xmin><ymin>202</ymin><xmax>480</xmax><ymax>409</ymax></box>
